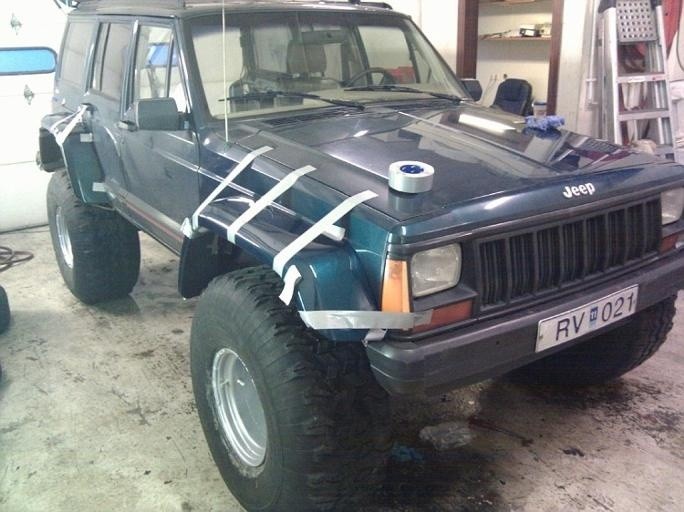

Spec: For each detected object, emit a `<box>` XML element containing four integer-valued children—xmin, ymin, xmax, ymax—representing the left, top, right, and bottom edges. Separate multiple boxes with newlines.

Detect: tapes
<box><xmin>387</xmin><ymin>160</ymin><xmax>435</xmax><ymax>195</ymax></box>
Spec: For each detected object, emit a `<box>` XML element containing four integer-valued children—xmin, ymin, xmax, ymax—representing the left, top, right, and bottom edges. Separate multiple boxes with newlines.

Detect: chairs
<box><xmin>275</xmin><ymin>39</ymin><xmax>340</xmax><ymax>105</ymax></box>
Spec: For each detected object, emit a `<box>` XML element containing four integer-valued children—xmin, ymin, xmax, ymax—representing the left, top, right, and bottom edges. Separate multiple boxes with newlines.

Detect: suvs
<box><xmin>32</xmin><ymin>2</ymin><xmax>683</xmax><ymax>512</ymax></box>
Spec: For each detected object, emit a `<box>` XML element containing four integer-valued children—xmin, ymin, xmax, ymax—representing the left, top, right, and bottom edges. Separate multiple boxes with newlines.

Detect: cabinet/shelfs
<box><xmin>456</xmin><ymin>0</ymin><xmax>563</xmax><ymax>117</ymax></box>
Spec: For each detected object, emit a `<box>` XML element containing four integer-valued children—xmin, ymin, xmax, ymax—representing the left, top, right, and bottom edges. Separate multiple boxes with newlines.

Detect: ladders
<box><xmin>593</xmin><ymin>0</ymin><xmax>679</xmax><ymax>167</ymax></box>
